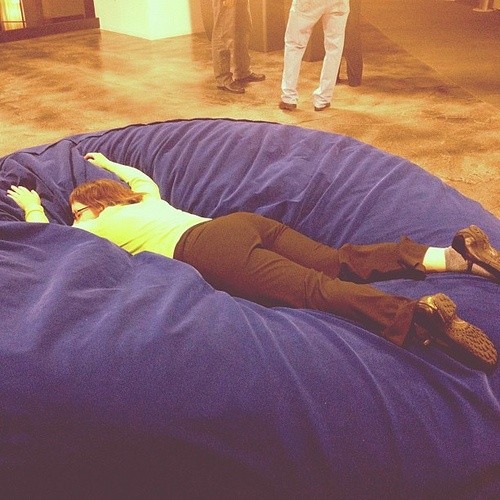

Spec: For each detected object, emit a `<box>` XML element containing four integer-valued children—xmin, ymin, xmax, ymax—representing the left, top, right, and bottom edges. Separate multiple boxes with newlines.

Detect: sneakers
<box><xmin>452</xmin><ymin>225</ymin><xmax>500</xmax><ymax>280</ymax></box>
<box><xmin>413</xmin><ymin>292</ymin><xmax>497</xmax><ymax>374</ymax></box>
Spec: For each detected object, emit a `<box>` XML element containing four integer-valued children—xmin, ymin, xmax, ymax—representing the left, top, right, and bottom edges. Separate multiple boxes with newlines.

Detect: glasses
<box><xmin>74</xmin><ymin>204</ymin><xmax>92</xmax><ymax>219</ymax></box>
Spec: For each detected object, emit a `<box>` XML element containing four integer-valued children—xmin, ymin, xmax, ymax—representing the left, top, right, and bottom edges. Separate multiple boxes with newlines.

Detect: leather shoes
<box><xmin>236</xmin><ymin>73</ymin><xmax>265</xmax><ymax>82</ymax></box>
<box><xmin>217</xmin><ymin>82</ymin><xmax>245</xmax><ymax>93</ymax></box>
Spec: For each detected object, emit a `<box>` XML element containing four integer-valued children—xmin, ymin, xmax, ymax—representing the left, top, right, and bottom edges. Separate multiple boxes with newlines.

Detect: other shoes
<box><xmin>314</xmin><ymin>103</ymin><xmax>330</xmax><ymax>111</ymax></box>
<box><xmin>279</xmin><ymin>102</ymin><xmax>297</xmax><ymax>111</ymax></box>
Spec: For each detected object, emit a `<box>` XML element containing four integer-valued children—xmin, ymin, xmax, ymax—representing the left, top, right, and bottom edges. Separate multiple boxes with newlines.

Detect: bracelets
<box><xmin>24</xmin><ymin>205</ymin><xmax>44</xmax><ymax>217</ymax></box>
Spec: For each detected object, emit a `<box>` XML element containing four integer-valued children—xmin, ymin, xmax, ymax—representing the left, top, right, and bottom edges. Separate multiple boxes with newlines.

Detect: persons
<box><xmin>209</xmin><ymin>0</ymin><xmax>267</xmax><ymax>94</ymax></box>
<box><xmin>330</xmin><ymin>0</ymin><xmax>364</xmax><ymax>87</ymax></box>
<box><xmin>278</xmin><ymin>0</ymin><xmax>351</xmax><ymax>111</ymax></box>
<box><xmin>7</xmin><ymin>151</ymin><xmax>500</xmax><ymax>368</ymax></box>
